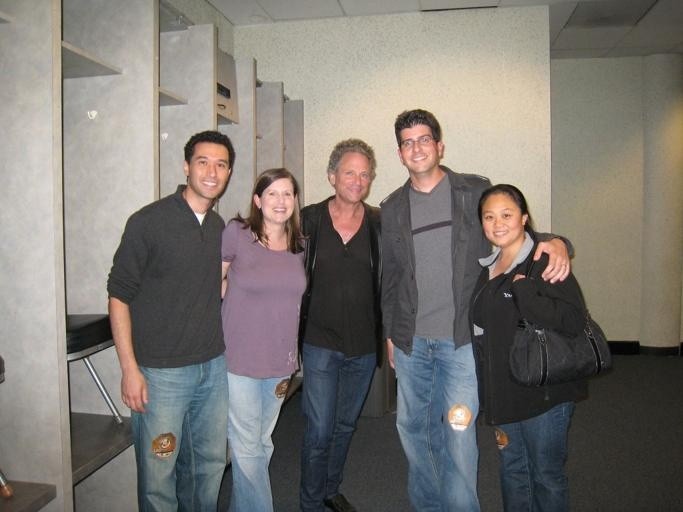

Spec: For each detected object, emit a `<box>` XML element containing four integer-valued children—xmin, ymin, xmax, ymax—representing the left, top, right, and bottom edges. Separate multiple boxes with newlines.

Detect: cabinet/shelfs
<box><xmin>0</xmin><ymin>0</ymin><xmax>305</xmax><ymax>511</ymax></box>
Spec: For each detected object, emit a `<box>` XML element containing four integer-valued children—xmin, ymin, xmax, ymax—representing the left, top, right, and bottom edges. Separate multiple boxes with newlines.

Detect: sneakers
<box><xmin>323</xmin><ymin>490</ymin><xmax>356</xmax><ymax>511</ymax></box>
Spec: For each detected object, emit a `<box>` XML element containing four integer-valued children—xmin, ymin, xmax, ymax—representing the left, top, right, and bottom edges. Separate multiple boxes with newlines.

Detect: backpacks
<box><xmin>503</xmin><ymin>254</ymin><xmax>613</xmax><ymax>386</ymax></box>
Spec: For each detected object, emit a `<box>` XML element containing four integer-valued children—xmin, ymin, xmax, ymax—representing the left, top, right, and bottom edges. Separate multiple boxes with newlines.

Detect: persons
<box><xmin>291</xmin><ymin>138</ymin><xmax>383</xmax><ymax>511</ymax></box>
<box><xmin>470</xmin><ymin>183</ymin><xmax>588</xmax><ymax>512</ymax></box>
<box><xmin>219</xmin><ymin>166</ymin><xmax>308</xmax><ymax>512</ymax></box>
<box><xmin>106</xmin><ymin>130</ymin><xmax>236</xmax><ymax>512</ymax></box>
<box><xmin>379</xmin><ymin>110</ymin><xmax>576</xmax><ymax>512</ymax></box>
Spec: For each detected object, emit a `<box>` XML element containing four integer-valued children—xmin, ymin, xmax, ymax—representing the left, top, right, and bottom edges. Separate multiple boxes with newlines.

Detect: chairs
<box><xmin>1</xmin><ymin>356</ymin><xmax>15</xmax><ymax>499</ymax></box>
<box><xmin>66</xmin><ymin>314</ymin><xmax>123</xmax><ymax>425</ymax></box>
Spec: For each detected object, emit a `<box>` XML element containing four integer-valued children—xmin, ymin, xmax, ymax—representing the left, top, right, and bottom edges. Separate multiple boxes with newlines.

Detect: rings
<box><xmin>562</xmin><ymin>264</ymin><xmax>567</xmax><ymax>267</ymax></box>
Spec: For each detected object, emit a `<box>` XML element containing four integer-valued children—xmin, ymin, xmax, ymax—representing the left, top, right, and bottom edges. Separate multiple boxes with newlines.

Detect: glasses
<box><xmin>403</xmin><ymin>134</ymin><xmax>438</xmax><ymax>146</ymax></box>
